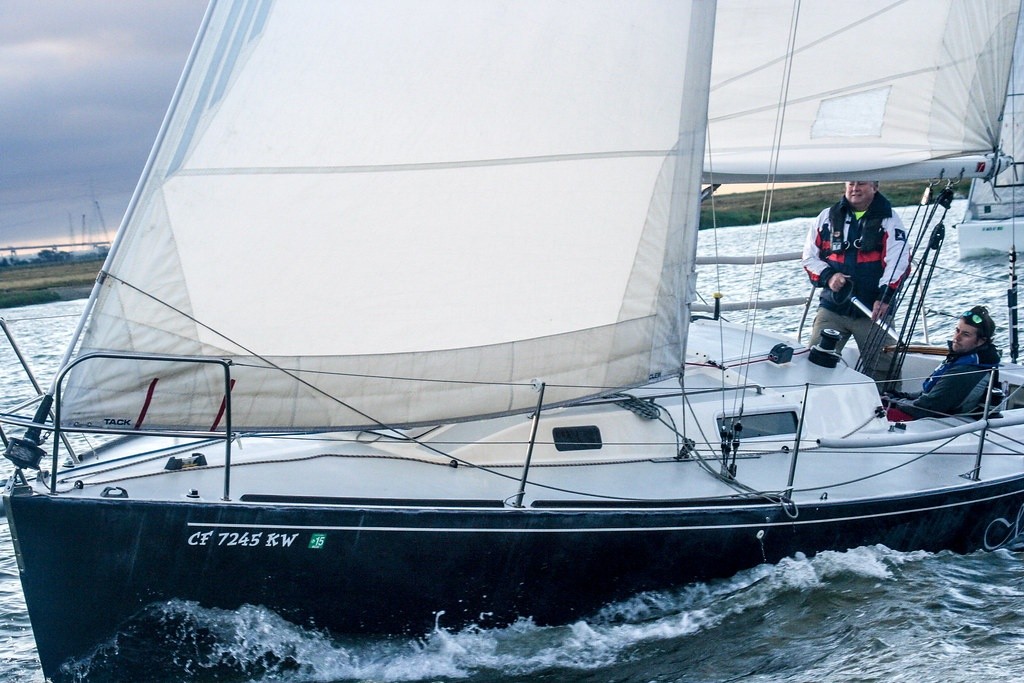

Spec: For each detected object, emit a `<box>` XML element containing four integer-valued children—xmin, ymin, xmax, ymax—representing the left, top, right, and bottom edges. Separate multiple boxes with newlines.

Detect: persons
<box><xmin>801</xmin><ymin>180</ymin><xmax>910</xmax><ymax>394</ymax></box>
<box><xmin>880</xmin><ymin>306</ymin><xmax>1004</xmax><ymax>422</ymax></box>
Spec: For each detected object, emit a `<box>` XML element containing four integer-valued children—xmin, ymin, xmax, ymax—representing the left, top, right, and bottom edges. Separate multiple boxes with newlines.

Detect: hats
<box><xmin>962</xmin><ymin>306</ymin><xmax>996</xmax><ymax>338</ymax></box>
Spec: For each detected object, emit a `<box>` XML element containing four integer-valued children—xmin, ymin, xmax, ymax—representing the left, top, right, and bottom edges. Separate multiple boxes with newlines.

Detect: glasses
<box><xmin>962</xmin><ymin>311</ymin><xmax>986</xmax><ymax>327</ymax></box>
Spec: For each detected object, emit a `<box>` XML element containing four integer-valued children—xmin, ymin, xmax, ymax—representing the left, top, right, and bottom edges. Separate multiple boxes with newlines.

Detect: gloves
<box><xmin>881</xmin><ymin>389</ymin><xmax>906</xmax><ymax>399</ymax></box>
<box><xmin>881</xmin><ymin>396</ymin><xmax>897</xmax><ymax>409</ymax></box>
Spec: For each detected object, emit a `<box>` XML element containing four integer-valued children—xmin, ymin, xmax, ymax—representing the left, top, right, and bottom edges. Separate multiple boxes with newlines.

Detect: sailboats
<box><xmin>952</xmin><ymin>10</ymin><xmax>1024</xmax><ymax>262</ymax></box>
<box><xmin>0</xmin><ymin>1</ymin><xmax>1024</xmax><ymax>683</ymax></box>
